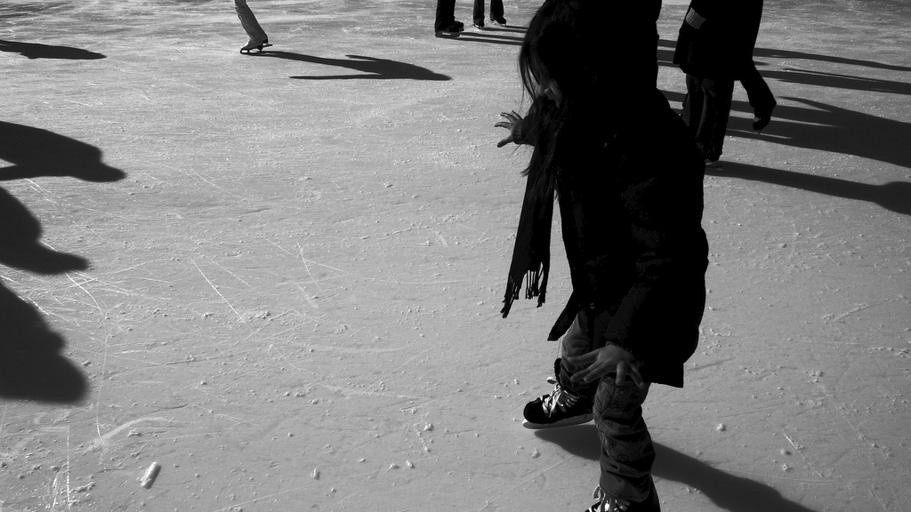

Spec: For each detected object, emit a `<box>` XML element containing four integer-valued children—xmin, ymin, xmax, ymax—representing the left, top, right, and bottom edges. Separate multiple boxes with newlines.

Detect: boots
<box><xmin>523</xmin><ymin>358</ymin><xmax>594</xmax><ymax>424</ymax></box>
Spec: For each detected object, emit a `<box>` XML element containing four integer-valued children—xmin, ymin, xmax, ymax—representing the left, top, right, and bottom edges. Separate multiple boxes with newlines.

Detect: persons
<box><xmin>733</xmin><ymin>54</ymin><xmax>777</xmax><ymax>135</ymax></box>
<box><xmin>473</xmin><ymin>0</ymin><xmax>508</xmax><ymax>27</ymax></box>
<box><xmin>434</xmin><ymin>1</ymin><xmax>464</xmax><ymax>34</ymax></box>
<box><xmin>492</xmin><ymin>0</ymin><xmax>712</xmax><ymax>512</ymax></box>
<box><xmin>234</xmin><ymin>1</ymin><xmax>268</xmax><ymax>50</ymax></box>
<box><xmin>673</xmin><ymin>0</ymin><xmax>767</xmax><ymax>164</ymax></box>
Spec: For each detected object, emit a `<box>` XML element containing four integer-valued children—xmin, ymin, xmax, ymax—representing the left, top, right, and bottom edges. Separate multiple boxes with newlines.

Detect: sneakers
<box><xmin>242</xmin><ymin>34</ymin><xmax>268</xmax><ymax>50</ymax></box>
<box><xmin>753</xmin><ymin>98</ymin><xmax>776</xmax><ymax>131</ymax></box>
<box><xmin>473</xmin><ymin>14</ymin><xmax>506</xmax><ymax>27</ymax></box>
<box><xmin>435</xmin><ymin>21</ymin><xmax>463</xmax><ymax>32</ymax></box>
